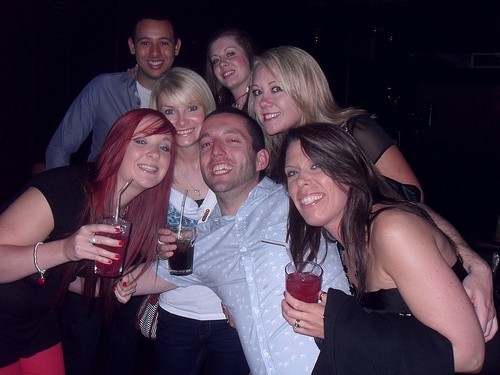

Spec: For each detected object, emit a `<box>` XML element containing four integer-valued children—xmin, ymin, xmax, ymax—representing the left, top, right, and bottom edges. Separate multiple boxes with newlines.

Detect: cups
<box><xmin>285</xmin><ymin>261</ymin><xmax>323</xmax><ymax>309</ymax></box>
<box><xmin>94</xmin><ymin>217</ymin><xmax>131</xmax><ymax>276</ymax></box>
<box><xmin>166</xmin><ymin>225</ymin><xmax>194</xmax><ymax>276</ymax></box>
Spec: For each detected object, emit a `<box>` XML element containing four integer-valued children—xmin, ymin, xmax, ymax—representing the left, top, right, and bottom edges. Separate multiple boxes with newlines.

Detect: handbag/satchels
<box><xmin>135</xmin><ymin>294</ymin><xmax>158</xmax><ymax>341</ymax></box>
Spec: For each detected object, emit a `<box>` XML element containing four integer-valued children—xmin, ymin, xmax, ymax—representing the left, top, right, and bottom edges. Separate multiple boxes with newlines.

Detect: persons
<box><xmin>0</xmin><ymin>17</ymin><xmax>500</xmax><ymax>375</ymax></box>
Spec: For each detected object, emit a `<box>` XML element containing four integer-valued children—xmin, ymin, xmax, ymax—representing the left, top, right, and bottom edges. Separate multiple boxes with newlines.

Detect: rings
<box><xmin>157</xmin><ymin>251</ymin><xmax>163</xmax><ymax>259</ymax></box>
<box><xmin>90</xmin><ymin>235</ymin><xmax>96</xmax><ymax>244</ymax></box>
<box><xmin>157</xmin><ymin>237</ymin><xmax>164</xmax><ymax>246</ymax></box>
<box><xmin>295</xmin><ymin>319</ymin><xmax>300</xmax><ymax>327</ymax></box>
<box><xmin>319</xmin><ymin>292</ymin><xmax>326</xmax><ymax>302</ymax></box>
<box><xmin>226</xmin><ymin>315</ymin><xmax>229</xmax><ymax>318</ymax></box>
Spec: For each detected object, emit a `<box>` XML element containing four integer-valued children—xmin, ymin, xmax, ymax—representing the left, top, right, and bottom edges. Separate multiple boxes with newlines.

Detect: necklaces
<box><xmin>233</xmin><ymin>92</ymin><xmax>248</xmax><ymax>102</ymax></box>
<box><xmin>121</xmin><ymin>205</ymin><xmax>129</xmax><ymax>219</ymax></box>
<box><xmin>174</xmin><ymin>160</ymin><xmax>203</xmax><ymax>197</ymax></box>
<box><xmin>341</xmin><ymin>248</ymin><xmax>359</xmax><ymax>295</ymax></box>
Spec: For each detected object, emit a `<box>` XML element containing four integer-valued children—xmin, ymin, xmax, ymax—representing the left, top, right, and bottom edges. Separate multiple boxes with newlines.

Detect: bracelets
<box><xmin>34</xmin><ymin>241</ymin><xmax>47</xmax><ymax>283</ymax></box>
